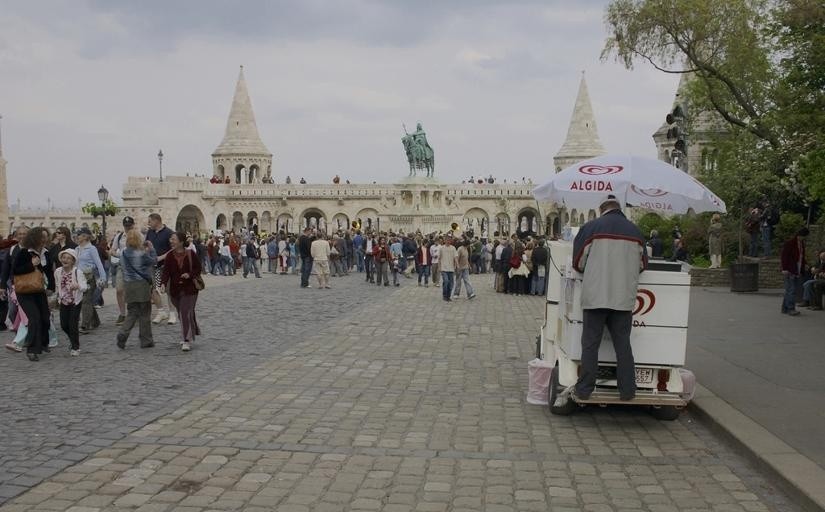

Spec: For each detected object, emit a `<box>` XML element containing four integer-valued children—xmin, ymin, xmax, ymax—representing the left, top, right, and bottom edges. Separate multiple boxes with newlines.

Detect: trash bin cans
<box><xmin>728</xmin><ymin>261</ymin><xmax>759</xmax><ymax>292</ymax></box>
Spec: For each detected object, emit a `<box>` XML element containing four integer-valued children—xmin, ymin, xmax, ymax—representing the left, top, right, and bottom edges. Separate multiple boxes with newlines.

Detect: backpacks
<box><xmin>13</xmin><ymin>267</ymin><xmax>45</xmax><ymax>293</ymax></box>
<box><xmin>510</xmin><ymin>250</ymin><xmax>522</xmax><ymax>269</ymax></box>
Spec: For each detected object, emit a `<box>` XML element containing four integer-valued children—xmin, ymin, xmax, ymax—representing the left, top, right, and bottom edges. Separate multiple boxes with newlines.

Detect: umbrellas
<box><xmin>530</xmin><ymin>152</ymin><xmax>727</xmax><ymax>216</ymax></box>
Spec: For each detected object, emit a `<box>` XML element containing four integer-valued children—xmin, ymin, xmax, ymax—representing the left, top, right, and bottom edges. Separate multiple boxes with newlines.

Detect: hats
<box><xmin>122</xmin><ymin>216</ymin><xmax>135</xmax><ymax>228</ymax></box>
<box><xmin>57</xmin><ymin>248</ymin><xmax>77</xmax><ymax>265</ymax></box>
<box><xmin>599</xmin><ymin>192</ymin><xmax>620</xmax><ymax>208</ymax></box>
<box><xmin>77</xmin><ymin>227</ymin><xmax>91</xmax><ymax>236</ymax></box>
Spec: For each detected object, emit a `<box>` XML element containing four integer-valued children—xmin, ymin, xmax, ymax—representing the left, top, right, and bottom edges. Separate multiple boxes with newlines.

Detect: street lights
<box><xmin>155</xmin><ymin>149</ymin><xmax>164</xmax><ymax>187</ymax></box>
<box><xmin>97</xmin><ymin>184</ymin><xmax>111</xmax><ymax>253</ymax></box>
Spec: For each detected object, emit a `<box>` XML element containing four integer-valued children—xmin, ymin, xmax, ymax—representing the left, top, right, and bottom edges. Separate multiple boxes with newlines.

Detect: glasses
<box><xmin>55</xmin><ymin>231</ymin><xmax>63</xmax><ymax>235</ymax></box>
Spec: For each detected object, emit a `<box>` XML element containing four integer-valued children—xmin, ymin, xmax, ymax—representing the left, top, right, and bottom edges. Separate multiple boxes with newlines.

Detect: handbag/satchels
<box><xmin>187</xmin><ymin>248</ymin><xmax>205</xmax><ymax>290</ymax></box>
<box><xmin>110</xmin><ymin>263</ymin><xmax>118</xmax><ymax>275</ymax></box>
<box><xmin>151</xmin><ymin>288</ymin><xmax>163</xmax><ymax>309</ymax></box>
<box><xmin>253</xmin><ymin>249</ymin><xmax>259</xmax><ymax>258</ymax></box>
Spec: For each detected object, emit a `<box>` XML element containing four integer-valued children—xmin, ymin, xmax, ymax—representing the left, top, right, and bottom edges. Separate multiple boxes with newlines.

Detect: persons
<box><xmin>460</xmin><ymin>174</ymin><xmax>533</xmax><ymax>184</ymax></box>
<box><xmin>643</xmin><ymin>200</ymin><xmax>825</xmax><ymax>316</ymax></box>
<box><xmin>572</xmin><ymin>195</ymin><xmax>649</xmax><ymax>401</ymax></box>
<box><xmin>332</xmin><ymin>174</ymin><xmax>341</xmax><ymax>184</ymax></box>
<box><xmin>187</xmin><ymin>172</ymin><xmax>308</xmax><ymax>184</ymax></box>
<box><xmin>1</xmin><ymin>212</ymin><xmax>552</xmax><ymax>361</ymax></box>
<box><xmin>406</xmin><ymin>122</ymin><xmax>427</xmax><ymax>160</ymax></box>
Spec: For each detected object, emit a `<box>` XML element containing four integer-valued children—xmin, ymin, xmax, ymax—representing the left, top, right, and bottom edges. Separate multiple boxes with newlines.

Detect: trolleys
<box><xmin>530</xmin><ymin>242</ymin><xmax>691</xmax><ymax>422</ymax></box>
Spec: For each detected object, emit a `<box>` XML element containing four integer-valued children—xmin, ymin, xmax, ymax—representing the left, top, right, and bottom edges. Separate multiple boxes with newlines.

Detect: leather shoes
<box><xmin>27</xmin><ymin>352</ymin><xmax>40</xmax><ymax>361</ymax></box>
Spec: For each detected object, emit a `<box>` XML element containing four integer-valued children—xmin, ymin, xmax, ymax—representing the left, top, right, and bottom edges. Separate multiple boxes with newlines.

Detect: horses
<box><xmin>401</xmin><ymin>134</ymin><xmax>434</xmax><ymax>178</ymax></box>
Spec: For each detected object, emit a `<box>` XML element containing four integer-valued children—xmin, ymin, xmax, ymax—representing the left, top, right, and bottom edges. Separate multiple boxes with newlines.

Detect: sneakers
<box><xmin>167</xmin><ymin>311</ymin><xmax>178</xmax><ymax>325</ymax></box>
<box><xmin>794</xmin><ymin>296</ymin><xmax>822</xmax><ymax>310</ymax></box>
<box><xmin>781</xmin><ymin>305</ymin><xmax>800</xmax><ymax>316</ymax></box>
<box><xmin>207</xmin><ymin>271</ymin><xmax>476</xmax><ymax>301</ymax></box>
<box><xmin>180</xmin><ymin>341</ymin><xmax>192</xmax><ymax>351</ymax></box>
<box><xmin>117</xmin><ymin>334</ymin><xmax>127</xmax><ymax>350</ymax></box>
<box><xmin>48</xmin><ymin>338</ymin><xmax>58</xmax><ymax>348</ymax></box>
<box><xmin>151</xmin><ymin>310</ymin><xmax>168</xmax><ymax>324</ymax></box>
<box><xmin>140</xmin><ymin>339</ymin><xmax>155</xmax><ymax>348</ymax></box>
<box><xmin>79</xmin><ymin>327</ymin><xmax>91</xmax><ymax>333</ymax></box>
<box><xmin>115</xmin><ymin>314</ymin><xmax>125</xmax><ymax>324</ymax></box>
<box><xmin>4</xmin><ymin>343</ymin><xmax>23</xmax><ymax>352</ymax></box>
<box><xmin>66</xmin><ymin>339</ymin><xmax>81</xmax><ymax>357</ymax></box>
